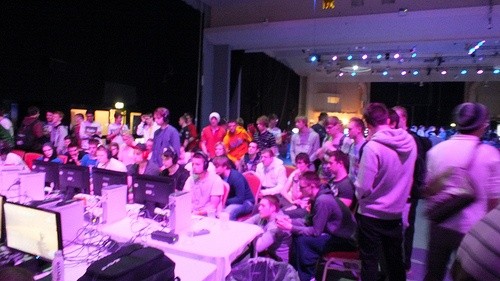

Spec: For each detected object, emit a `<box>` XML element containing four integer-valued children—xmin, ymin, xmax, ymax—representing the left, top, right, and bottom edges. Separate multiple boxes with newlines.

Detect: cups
<box><xmin>220</xmin><ymin>210</ymin><xmax>230</xmax><ymax>230</ymax></box>
<box><xmin>206</xmin><ymin>207</ymin><xmax>216</xmax><ymax>223</ymax></box>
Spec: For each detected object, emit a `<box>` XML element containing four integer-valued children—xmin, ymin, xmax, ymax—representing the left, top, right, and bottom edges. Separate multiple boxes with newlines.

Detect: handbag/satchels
<box><xmin>423</xmin><ymin>167</ymin><xmax>477</xmax><ymax>223</ymax></box>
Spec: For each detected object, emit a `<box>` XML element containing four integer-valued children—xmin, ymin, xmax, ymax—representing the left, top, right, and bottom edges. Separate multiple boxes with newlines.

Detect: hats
<box><xmin>209</xmin><ymin>112</ymin><xmax>220</xmax><ymax>123</ymax></box>
<box><xmin>453</xmin><ymin>102</ymin><xmax>488</xmax><ymax>130</ymax></box>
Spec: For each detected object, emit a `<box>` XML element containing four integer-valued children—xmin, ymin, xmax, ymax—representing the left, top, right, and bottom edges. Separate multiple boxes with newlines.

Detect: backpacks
<box><xmin>15</xmin><ymin>119</ymin><xmax>40</xmax><ymax>149</ymax></box>
<box><xmin>76</xmin><ymin>244</ymin><xmax>181</xmax><ymax>281</ymax></box>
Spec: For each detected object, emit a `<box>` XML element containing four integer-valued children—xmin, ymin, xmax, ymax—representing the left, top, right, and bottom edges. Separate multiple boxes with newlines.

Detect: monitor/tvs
<box><xmin>91</xmin><ymin>167</ymin><xmax>127</xmax><ymax>198</ymax></box>
<box><xmin>33</xmin><ymin>160</ymin><xmax>60</xmax><ymax>193</ymax></box>
<box><xmin>3</xmin><ymin>202</ymin><xmax>62</xmax><ymax>273</ymax></box>
<box><xmin>131</xmin><ymin>173</ymin><xmax>176</xmax><ymax>223</ymax></box>
<box><xmin>57</xmin><ymin>165</ymin><xmax>89</xmax><ymax>206</ymax></box>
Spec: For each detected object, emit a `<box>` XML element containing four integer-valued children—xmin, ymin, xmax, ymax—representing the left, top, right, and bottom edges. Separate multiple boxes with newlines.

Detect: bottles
<box><xmin>52</xmin><ymin>250</ymin><xmax>64</xmax><ymax>281</ymax></box>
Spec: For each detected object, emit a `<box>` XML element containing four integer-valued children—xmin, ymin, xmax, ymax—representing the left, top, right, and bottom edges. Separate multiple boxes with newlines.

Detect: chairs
<box><xmin>13</xmin><ymin>150</ymin><xmax>68</xmax><ymax>169</ymax></box>
<box><xmin>197</xmin><ymin>164</ymin><xmax>363</xmax><ymax>281</ymax></box>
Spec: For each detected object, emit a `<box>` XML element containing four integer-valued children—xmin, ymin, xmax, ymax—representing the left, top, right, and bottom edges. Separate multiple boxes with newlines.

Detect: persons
<box><xmin>278</xmin><ymin>165</ymin><xmax>357</xmax><ymax>281</ymax></box>
<box><xmin>355</xmin><ymin>100</ymin><xmax>418</xmax><ymax>280</ymax></box>
<box><xmin>248</xmin><ymin>193</ymin><xmax>294</xmax><ymax>269</ymax></box>
<box><xmin>0</xmin><ymin>108</ymin><xmax>500</xmax><ymax>280</ymax></box>
<box><xmin>421</xmin><ymin>101</ymin><xmax>500</xmax><ymax>281</ymax></box>
<box><xmin>448</xmin><ymin>200</ymin><xmax>500</xmax><ymax>281</ymax></box>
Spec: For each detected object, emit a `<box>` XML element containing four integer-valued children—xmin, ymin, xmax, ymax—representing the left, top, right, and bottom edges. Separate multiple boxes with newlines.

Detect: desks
<box><xmin>64</xmin><ymin>235</ymin><xmax>217</xmax><ymax>280</ymax></box>
<box><xmin>85</xmin><ymin>204</ymin><xmax>264</xmax><ymax>281</ymax></box>
